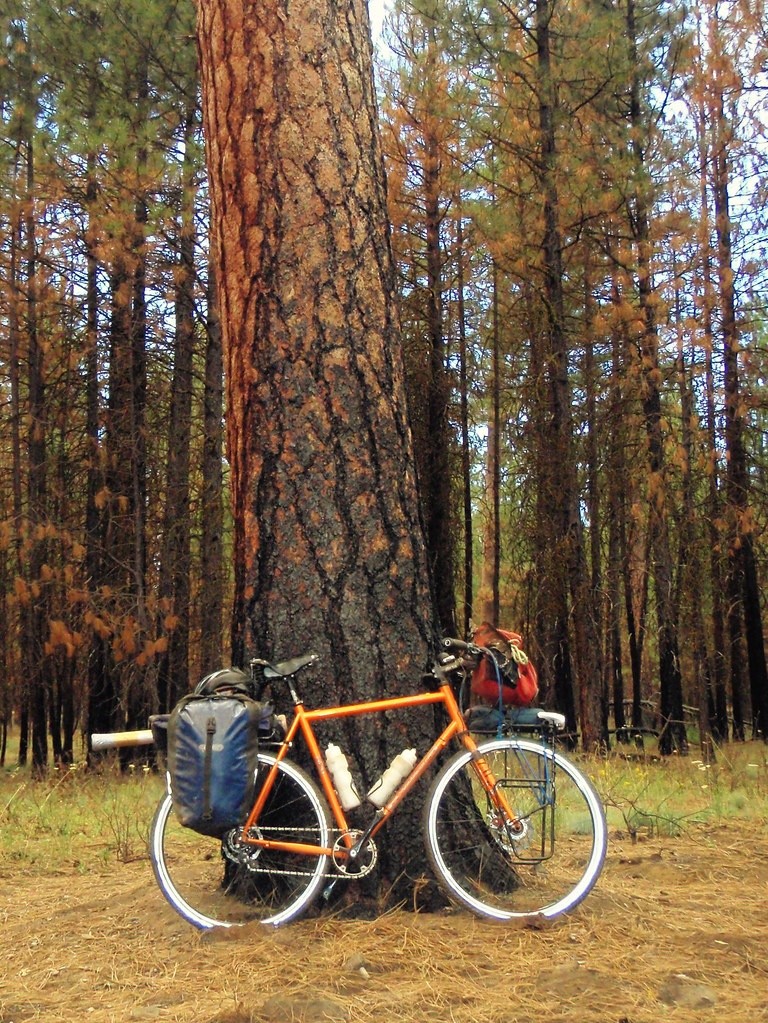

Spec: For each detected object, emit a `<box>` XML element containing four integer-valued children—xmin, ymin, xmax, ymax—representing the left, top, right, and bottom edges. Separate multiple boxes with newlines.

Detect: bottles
<box><xmin>325</xmin><ymin>742</ymin><xmax>362</xmax><ymax>813</ymax></box>
<box><xmin>366</xmin><ymin>747</ymin><xmax>419</xmax><ymax>810</ymax></box>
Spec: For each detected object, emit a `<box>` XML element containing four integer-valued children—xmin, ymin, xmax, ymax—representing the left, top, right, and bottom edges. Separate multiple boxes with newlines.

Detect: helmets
<box><xmin>195</xmin><ymin>667</ymin><xmax>255</xmax><ymax>696</ymax></box>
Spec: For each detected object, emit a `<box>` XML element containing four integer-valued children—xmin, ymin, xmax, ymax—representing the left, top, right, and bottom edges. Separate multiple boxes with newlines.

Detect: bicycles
<box><xmin>92</xmin><ymin>638</ymin><xmax>608</xmax><ymax>930</ymax></box>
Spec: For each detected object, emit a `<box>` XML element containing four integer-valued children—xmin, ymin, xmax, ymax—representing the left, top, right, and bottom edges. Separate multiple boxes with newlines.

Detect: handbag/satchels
<box><xmin>168</xmin><ymin>693</ymin><xmax>259</xmax><ymax>835</ymax></box>
<box><xmin>473</xmin><ymin>621</ymin><xmax>537</xmax><ymax>705</ymax></box>
